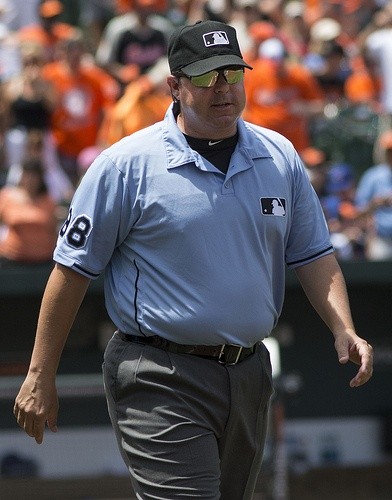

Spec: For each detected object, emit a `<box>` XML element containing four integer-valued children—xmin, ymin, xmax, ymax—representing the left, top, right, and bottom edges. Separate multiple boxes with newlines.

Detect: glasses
<box><xmin>183</xmin><ymin>66</ymin><xmax>243</xmax><ymax>87</ymax></box>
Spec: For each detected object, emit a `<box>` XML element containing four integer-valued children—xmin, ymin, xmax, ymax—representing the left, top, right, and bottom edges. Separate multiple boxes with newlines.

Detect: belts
<box><xmin>118</xmin><ymin>329</ymin><xmax>261</xmax><ymax>366</ymax></box>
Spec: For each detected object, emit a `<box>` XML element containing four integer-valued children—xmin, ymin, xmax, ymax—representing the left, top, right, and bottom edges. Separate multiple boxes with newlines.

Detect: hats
<box><xmin>168</xmin><ymin>21</ymin><xmax>253</xmax><ymax>76</ymax></box>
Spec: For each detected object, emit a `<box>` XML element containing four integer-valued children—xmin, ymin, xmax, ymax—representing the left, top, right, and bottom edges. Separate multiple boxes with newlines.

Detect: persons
<box><xmin>0</xmin><ymin>0</ymin><xmax>392</xmax><ymax>262</ymax></box>
<box><xmin>14</xmin><ymin>20</ymin><xmax>374</xmax><ymax>500</ymax></box>
